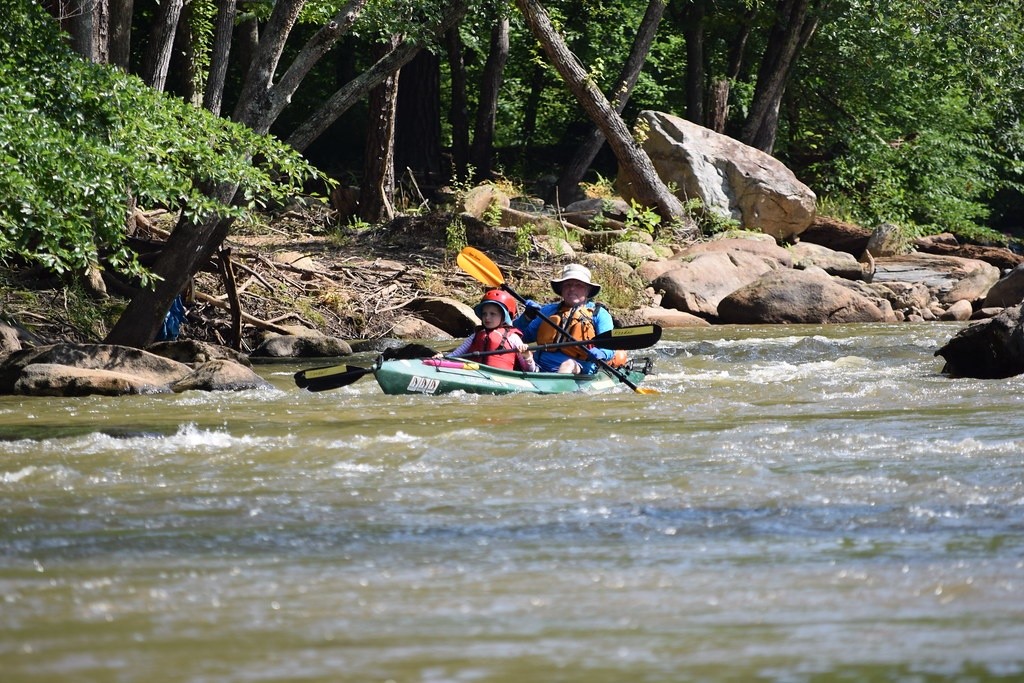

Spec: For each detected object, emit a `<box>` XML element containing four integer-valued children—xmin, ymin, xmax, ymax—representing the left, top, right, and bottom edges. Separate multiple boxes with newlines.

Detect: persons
<box><xmin>513</xmin><ymin>264</ymin><xmax>616</xmax><ymax>374</ymax></box>
<box><xmin>431</xmin><ymin>290</ymin><xmax>539</xmax><ymax>375</ymax></box>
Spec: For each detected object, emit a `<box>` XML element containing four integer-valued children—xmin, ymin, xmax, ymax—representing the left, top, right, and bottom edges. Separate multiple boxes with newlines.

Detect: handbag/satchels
<box><xmin>163</xmin><ymin>293</ymin><xmax>188</xmax><ymax>341</ymax></box>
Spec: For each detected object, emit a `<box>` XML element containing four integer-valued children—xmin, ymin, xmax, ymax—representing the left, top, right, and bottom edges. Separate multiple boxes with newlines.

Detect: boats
<box><xmin>370</xmin><ymin>343</ymin><xmax>654</xmax><ymax>395</ymax></box>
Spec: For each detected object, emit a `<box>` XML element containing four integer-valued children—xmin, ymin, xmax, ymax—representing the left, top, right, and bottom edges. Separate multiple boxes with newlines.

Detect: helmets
<box><xmin>480</xmin><ymin>289</ymin><xmax>516</xmax><ymax>319</ymax></box>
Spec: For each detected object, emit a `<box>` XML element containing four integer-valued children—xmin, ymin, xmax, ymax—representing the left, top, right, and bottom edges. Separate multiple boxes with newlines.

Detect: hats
<box><xmin>474</xmin><ymin>300</ymin><xmax>513</xmax><ymax>326</ymax></box>
<box><xmin>550</xmin><ymin>264</ymin><xmax>601</xmax><ymax>298</ymax></box>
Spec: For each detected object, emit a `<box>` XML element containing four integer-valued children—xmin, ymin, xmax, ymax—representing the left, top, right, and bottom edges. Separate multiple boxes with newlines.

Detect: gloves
<box><xmin>588</xmin><ymin>347</ymin><xmax>607</xmax><ymax>364</ymax></box>
<box><xmin>523</xmin><ymin>299</ymin><xmax>541</xmax><ymax>321</ymax></box>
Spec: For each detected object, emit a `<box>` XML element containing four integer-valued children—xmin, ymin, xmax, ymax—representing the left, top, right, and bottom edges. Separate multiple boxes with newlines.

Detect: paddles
<box><xmin>458</xmin><ymin>246</ymin><xmax>654</xmax><ymax>397</ymax></box>
<box><xmin>294</xmin><ymin>323</ymin><xmax>663</xmax><ymax>392</ymax></box>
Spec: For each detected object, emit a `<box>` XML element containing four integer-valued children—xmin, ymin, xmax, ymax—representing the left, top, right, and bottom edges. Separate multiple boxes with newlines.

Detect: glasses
<box><xmin>562</xmin><ymin>282</ymin><xmax>589</xmax><ymax>289</ymax></box>
